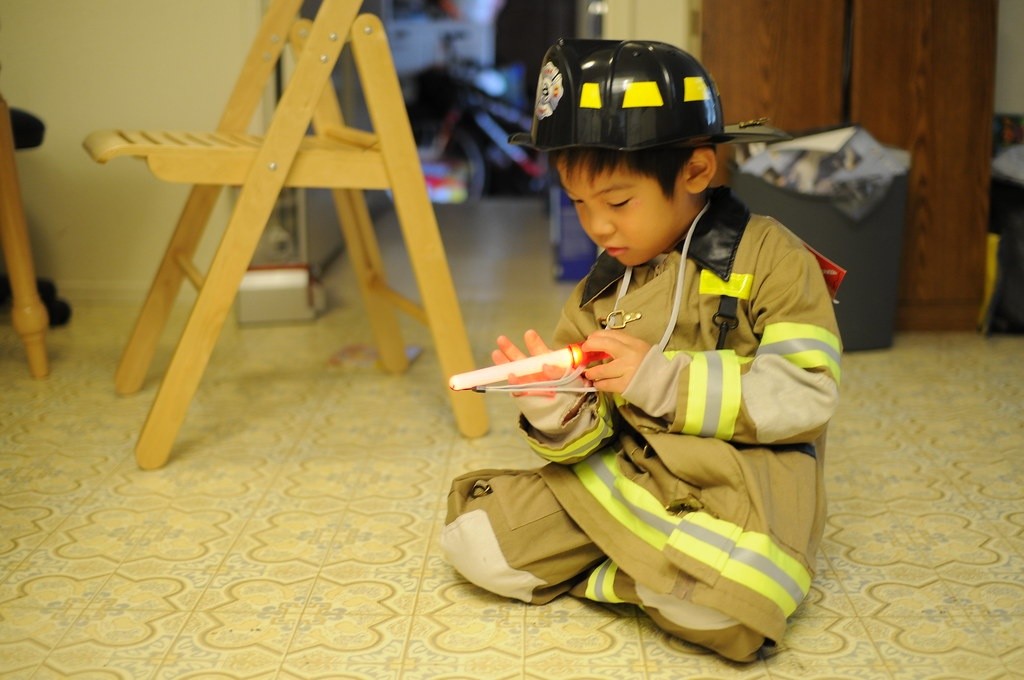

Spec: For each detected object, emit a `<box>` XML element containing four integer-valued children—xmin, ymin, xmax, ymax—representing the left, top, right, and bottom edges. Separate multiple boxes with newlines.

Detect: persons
<box><xmin>443</xmin><ymin>39</ymin><xmax>847</xmax><ymax>664</ymax></box>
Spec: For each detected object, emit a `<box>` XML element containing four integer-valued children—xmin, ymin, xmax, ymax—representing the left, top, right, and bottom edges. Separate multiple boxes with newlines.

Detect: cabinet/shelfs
<box><xmin>699</xmin><ymin>0</ymin><xmax>999</xmax><ymax>330</ymax></box>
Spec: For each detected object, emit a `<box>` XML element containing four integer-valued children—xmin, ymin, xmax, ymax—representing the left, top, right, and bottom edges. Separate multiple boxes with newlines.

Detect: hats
<box><xmin>507</xmin><ymin>37</ymin><xmax>794</xmax><ymax>153</ymax></box>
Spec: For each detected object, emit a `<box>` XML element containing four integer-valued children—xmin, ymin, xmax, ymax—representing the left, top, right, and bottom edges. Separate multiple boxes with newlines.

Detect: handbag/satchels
<box><xmin>728</xmin><ymin>125</ymin><xmax>907</xmax><ymax>352</ymax></box>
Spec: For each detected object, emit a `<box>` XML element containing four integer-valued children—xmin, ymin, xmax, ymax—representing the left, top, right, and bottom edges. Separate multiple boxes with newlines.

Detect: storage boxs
<box><xmin>726</xmin><ymin>122</ymin><xmax>913</xmax><ymax>352</ymax></box>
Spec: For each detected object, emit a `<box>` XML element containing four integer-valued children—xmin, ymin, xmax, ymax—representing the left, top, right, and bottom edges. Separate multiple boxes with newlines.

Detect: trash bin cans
<box><xmin>725</xmin><ymin>126</ymin><xmax>912</xmax><ymax>353</ymax></box>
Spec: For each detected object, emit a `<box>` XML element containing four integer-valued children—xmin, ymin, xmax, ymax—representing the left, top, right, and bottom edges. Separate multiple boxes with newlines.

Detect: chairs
<box><xmin>81</xmin><ymin>0</ymin><xmax>491</xmax><ymax>470</ymax></box>
